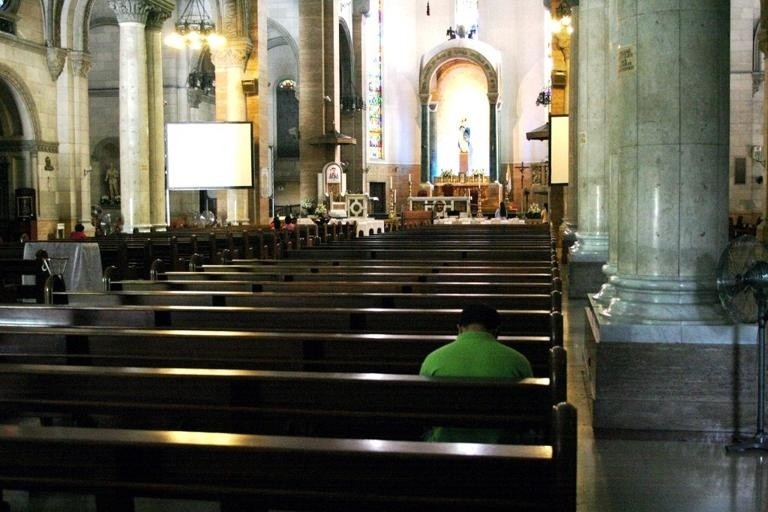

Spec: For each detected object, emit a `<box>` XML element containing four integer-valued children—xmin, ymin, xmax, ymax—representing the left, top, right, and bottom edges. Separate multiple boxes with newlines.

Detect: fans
<box><xmin>717</xmin><ymin>234</ymin><xmax>767</xmax><ymax>470</ymax></box>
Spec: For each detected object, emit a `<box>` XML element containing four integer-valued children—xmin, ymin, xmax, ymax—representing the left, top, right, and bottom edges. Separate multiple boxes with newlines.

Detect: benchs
<box><xmin>0</xmin><ymin>218</ymin><xmax>578</xmax><ymax>506</ymax></box>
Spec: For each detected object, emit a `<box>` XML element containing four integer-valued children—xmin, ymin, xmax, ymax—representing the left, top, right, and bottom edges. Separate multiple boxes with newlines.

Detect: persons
<box><xmin>329</xmin><ymin>167</ymin><xmax>338</xmax><ymax>179</ymax></box>
<box><xmin>495</xmin><ymin>202</ymin><xmax>508</xmax><ymax>219</ymax></box>
<box><xmin>70</xmin><ymin>223</ymin><xmax>87</xmax><ymax>239</ymax></box>
<box><xmin>104</xmin><ymin>162</ymin><xmax>120</xmax><ymax>205</ymax></box>
<box><xmin>419</xmin><ymin>303</ymin><xmax>534</xmax><ymax>444</ymax></box>
<box><xmin>541</xmin><ymin>202</ymin><xmax>549</xmax><ymax>224</ymax></box>
<box><xmin>271</xmin><ymin>215</ymin><xmax>296</xmax><ymax>233</ymax></box>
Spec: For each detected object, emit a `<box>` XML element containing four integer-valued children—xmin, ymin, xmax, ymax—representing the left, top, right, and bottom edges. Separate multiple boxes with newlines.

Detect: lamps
<box><xmin>162</xmin><ymin>1</ymin><xmax>228</xmax><ymax>52</ymax></box>
